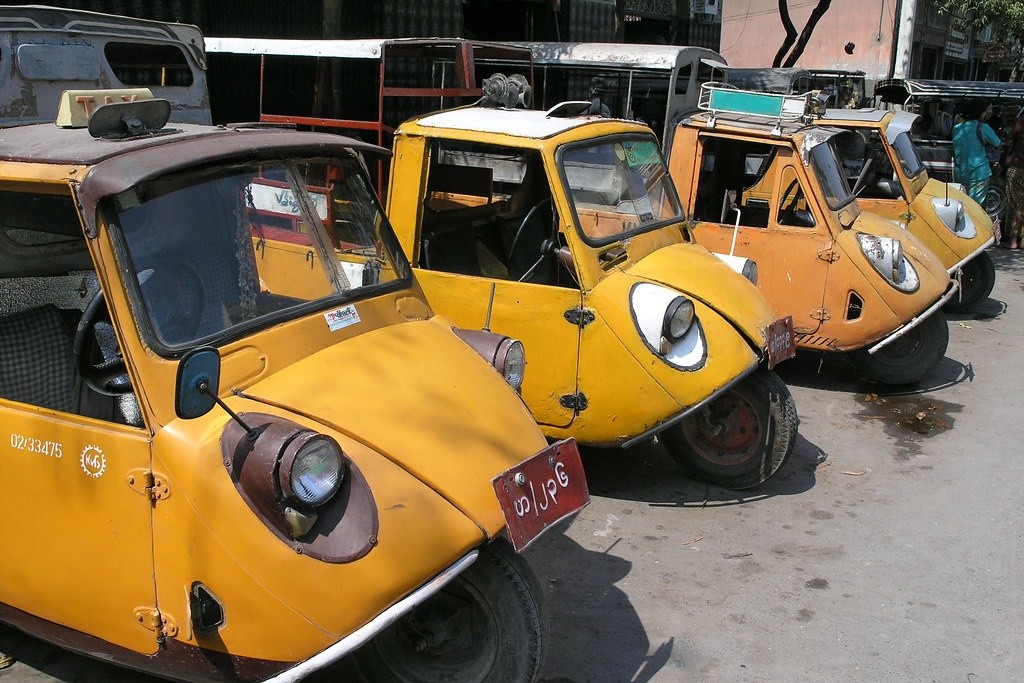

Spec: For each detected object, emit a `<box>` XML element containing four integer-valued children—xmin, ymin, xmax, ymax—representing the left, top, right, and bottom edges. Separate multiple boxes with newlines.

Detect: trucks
<box><xmin>467</xmin><ymin>39</ymin><xmax>1024</xmax><ymax>386</ymax></box>
<box><xmin>201</xmin><ymin>32</ymin><xmax>799</xmax><ymax>491</ymax></box>
<box><xmin>0</xmin><ymin>0</ymin><xmax>592</xmax><ymax>683</ymax></box>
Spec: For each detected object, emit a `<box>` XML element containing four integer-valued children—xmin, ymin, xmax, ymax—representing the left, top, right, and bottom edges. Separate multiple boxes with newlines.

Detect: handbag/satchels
<box><xmin>984</xmin><ymin>144</ymin><xmax>1002</xmax><ymax>163</ymax></box>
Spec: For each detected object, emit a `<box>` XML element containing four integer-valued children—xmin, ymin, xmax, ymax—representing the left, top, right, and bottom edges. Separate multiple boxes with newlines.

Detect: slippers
<box><xmin>997</xmin><ymin>242</ymin><xmax>1019</xmax><ymax>250</ymax></box>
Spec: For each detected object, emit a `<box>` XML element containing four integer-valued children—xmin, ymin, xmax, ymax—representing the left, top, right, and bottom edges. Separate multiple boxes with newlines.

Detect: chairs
<box><xmin>719</xmin><ymin>175</ymin><xmax>753</xmax><ymax>216</ymax></box>
<box><xmin>497</xmin><ymin>208</ymin><xmax>553</xmax><ymax>286</ymax></box>
<box><xmin>135</xmin><ymin>244</ymin><xmax>234</xmax><ymax>346</ymax></box>
<box><xmin>422</xmin><ymin>220</ymin><xmax>480</xmax><ymax>277</ymax></box>
<box><xmin>0</xmin><ymin>304</ymin><xmax>119</xmax><ymax>424</ymax></box>
<box><xmin>695</xmin><ymin>178</ymin><xmax>720</xmax><ymax>222</ymax></box>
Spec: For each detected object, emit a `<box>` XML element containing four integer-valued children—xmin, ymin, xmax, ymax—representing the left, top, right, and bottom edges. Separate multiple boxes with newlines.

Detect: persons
<box><xmin>579</xmin><ymin>78</ymin><xmax>611</xmax><ymax>118</ymax></box>
<box><xmin>951</xmin><ymin>98</ymin><xmax>1024</xmax><ymax>251</ymax></box>
